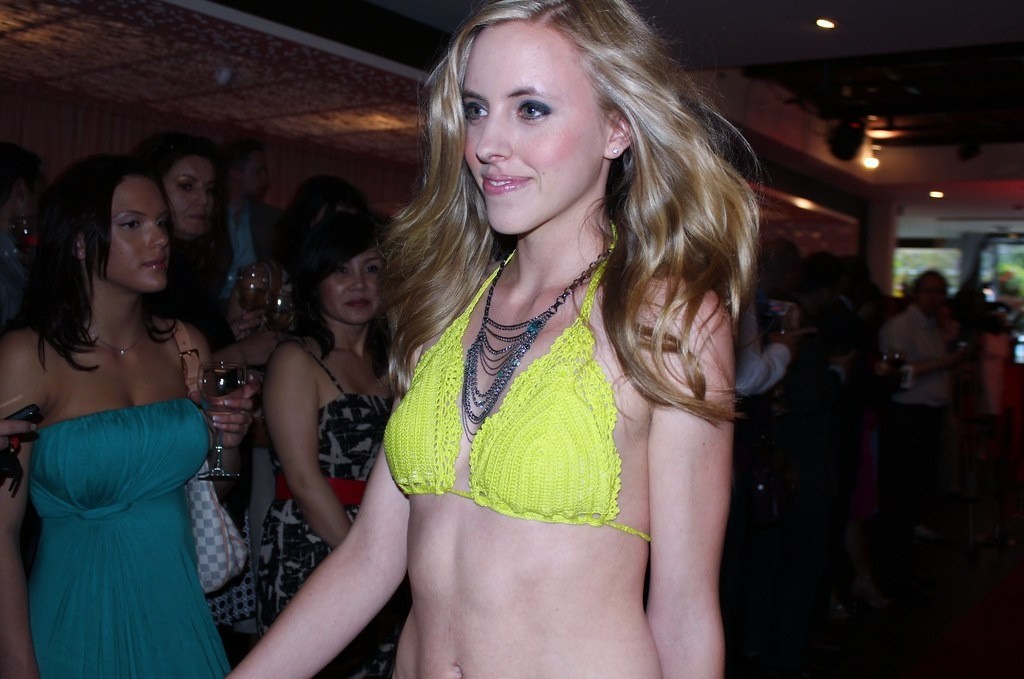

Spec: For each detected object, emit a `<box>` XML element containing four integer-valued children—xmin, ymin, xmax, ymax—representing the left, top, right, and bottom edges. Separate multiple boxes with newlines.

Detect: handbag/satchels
<box><xmin>164</xmin><ymin>319</ymin><xmax>247</xmax><ymax>592</ymax></box>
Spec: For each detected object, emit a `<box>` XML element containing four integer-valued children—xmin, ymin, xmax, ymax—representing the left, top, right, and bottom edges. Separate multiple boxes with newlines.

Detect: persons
<box><xmin>1</xmin><ymin>130</ymin><xmax>411</xmax><ymax>679</ymax></box>
<box><xmin>222</xmin><ymin>0</ymin><xmax>760</xmax><ymax>679</ymax></box>
<box><xmin>734</xmin><ymin>271</ymin><xmax>1012</xmax><ymax>630</ymax></box>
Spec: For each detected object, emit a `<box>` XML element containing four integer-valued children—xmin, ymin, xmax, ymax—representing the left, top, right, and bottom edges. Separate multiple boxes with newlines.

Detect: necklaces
<box><xmin>85</xmin><ymin>320</ymin><xmax>148</xmax><ymax>354</ymax></box>
<box><xmin>461</xmin><ymin>245</ymin><xmax>614</xmax><ymax>445</ymax></box>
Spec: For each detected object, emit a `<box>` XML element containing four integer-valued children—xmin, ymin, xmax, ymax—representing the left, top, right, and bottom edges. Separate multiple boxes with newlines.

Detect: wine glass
<box><xmin>196</xmin><ymin>361</ymin><xmax>247</xmax><ymax>479</ymax></box>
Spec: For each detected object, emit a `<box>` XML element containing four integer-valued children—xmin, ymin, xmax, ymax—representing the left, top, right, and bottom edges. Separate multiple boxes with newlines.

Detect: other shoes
<box><xmin>739</xmin><ymin>644</ymin><xmax>822</xmax><ymax>673</ymax></box>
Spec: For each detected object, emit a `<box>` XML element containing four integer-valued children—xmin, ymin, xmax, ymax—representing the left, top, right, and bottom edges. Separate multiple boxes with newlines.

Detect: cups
<box><xmin>886</xmin><ymin>348</ymin><xmax>905</xmax><ymax>371</ymax></box>
<box><xmin>10</xmin><ymin>218</ymin><xmax>39</xmax><ymax>251</ymax></box>
<box><xmin>770</xmin><ymin>325</ymin><xmax>793</xmax><ymax>335</ymax></box>
<box><xmin>236</xmin><ymin>262</ymin><xmax>272</xmax><ymax>312</ymax></box>
<box><xmin>264</xmin><ymin>291</ymin><xmax>297</xmax><ymax>332</ymax></box>
